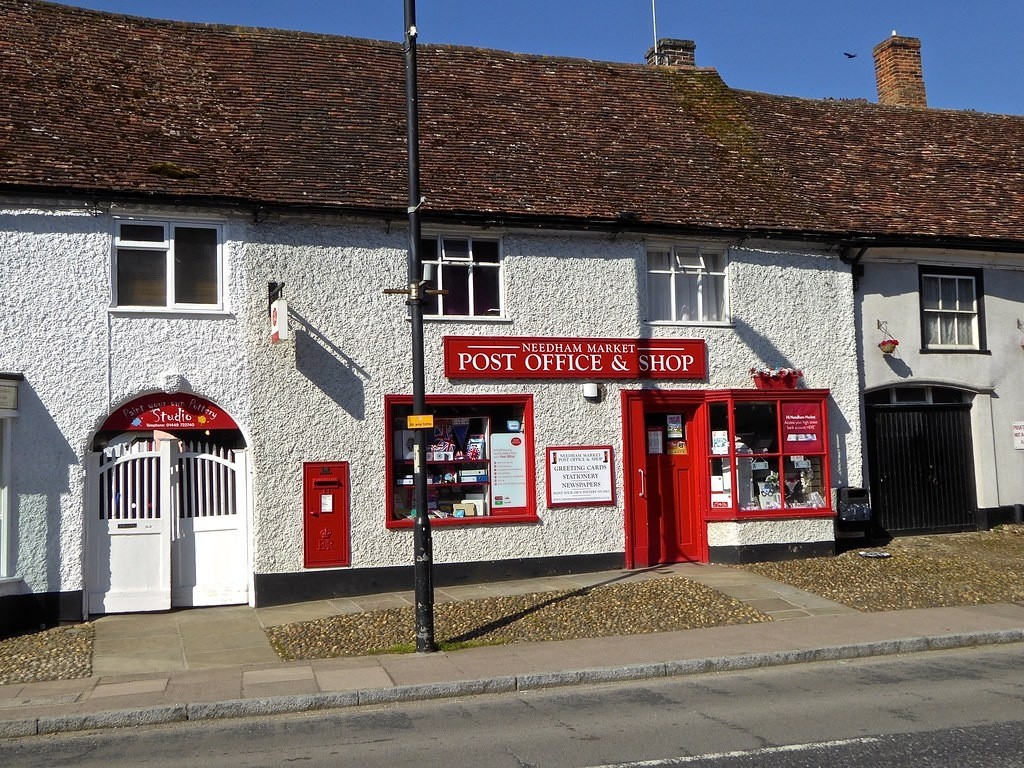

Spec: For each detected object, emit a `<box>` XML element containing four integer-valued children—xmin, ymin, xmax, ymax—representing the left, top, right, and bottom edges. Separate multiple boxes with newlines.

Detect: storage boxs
<box><xmin>394</xmin><ymin>430</ymin><xmax>414</xmax><ymax>461</ymax></box>
<box><xmin>453</xmin><ymin>470</ymin><xmax>488</xmax><ymax>516</ymax></box>
<box><xmin>394</xmin><ymin>478</ymin><xmax>414</xmax><ymax>486</ymax></box>
<box><xmin>427</xmin><ymin>452</ymin><xmax>453</xmax><ymax>461</ymax></box>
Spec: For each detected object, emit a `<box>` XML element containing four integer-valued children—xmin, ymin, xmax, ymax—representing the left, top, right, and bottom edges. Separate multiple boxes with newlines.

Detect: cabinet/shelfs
<box><xmin>393</xmin><ymin>416</ymin><xmax>491</xmax><ymax>516</ymax></box>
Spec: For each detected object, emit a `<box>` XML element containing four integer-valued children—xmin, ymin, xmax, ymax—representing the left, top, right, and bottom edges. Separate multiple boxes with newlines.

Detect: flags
<box><xmin>434</xmin><ymin>424</ymin><xmax>456</xmax><ymax>452</ymax></box>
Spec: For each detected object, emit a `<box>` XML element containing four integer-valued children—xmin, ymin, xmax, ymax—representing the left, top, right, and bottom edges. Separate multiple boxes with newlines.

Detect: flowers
<box><xmin>750</xmin><ymin>367</ymin><xmax>803</xmax><ymax>378</ymax></box>
<box><xmin>881</xmin><ymin>340</ymin><xmax>899</xmax><ymax>346</ymax></box>
